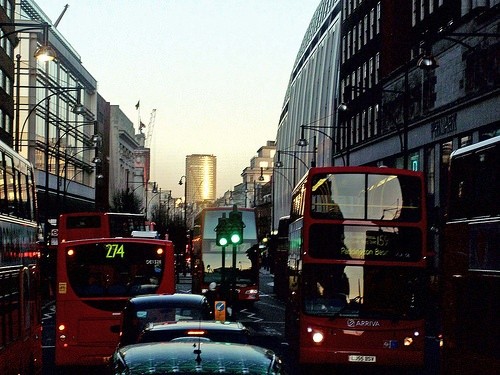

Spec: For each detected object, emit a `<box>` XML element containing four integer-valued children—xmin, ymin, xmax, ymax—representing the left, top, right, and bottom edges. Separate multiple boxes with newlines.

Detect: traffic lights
<box><xmin>229</xmin><ymin>211</ymin><xmax>243</xmax><ymax>245</ymax></box>
<box><xmin>216</xmin><ymin>218</ymin><xmax>229</xmax><ymax>246</ymax></box>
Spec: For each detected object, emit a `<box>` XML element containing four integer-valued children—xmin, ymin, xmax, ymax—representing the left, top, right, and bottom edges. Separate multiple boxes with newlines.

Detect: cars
<box><xmin>110</xmin><ymin>266</ymin><xmax>286</xmax><ymax>375</ymax></box>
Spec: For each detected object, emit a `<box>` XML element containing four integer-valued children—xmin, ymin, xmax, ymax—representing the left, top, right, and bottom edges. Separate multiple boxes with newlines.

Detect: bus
<box><xmin>275</xmin><ymin>215</ymin><xmax>291</xmax><ymax>304</ymax></box>
<box><xmin>0</xmin><ymin>139</ymin><xmax>47</xmax><ymax>375</ymax></box>
<box><xmin>189</xmin><ymin>204</ymin><xmax>262</xmax><ymax>303</ymax></box>
<box><xmin>432</xmin><ymin>133</ymin><xmax>500</xmax><ymax>375</ymax></box>
<box><xmin>283</xmin><ymin>165</ymin><xmax>438</xmax><ymax>375</ymax></box>
<box><xmin>53</xmin><ymin>234</ymin><xmax>177</xmax><ymax>368</ymax></box>
<box><xmin>57</xmin><ymin>213</ymin><xmax>157</xmax><ymax>240</ymax></box>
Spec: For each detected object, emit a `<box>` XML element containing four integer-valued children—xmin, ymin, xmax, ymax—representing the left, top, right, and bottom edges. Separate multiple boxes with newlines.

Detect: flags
<box><xmin>135</xmin><ymin>102</ymin><xmax>139</xmax><ymax>110</ymax></box>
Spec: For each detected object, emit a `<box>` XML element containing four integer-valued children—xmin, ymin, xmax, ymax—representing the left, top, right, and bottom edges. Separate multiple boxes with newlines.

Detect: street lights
<box><xmin>9</xmin><ymin>52</ymin><xmax>105</xmax><ymax>221</ymax></box>
<box><xmin>145</xmin><ymin>186</ymin><xmax>173</xmax><ymax>221</ymax></box>
<box><xmin>258</xmin><ymin>152</ymin><xmax>297</xmax><ymax>191</ymax></box>
<box><xmin>178</xmin><ymin>176</ymin><xmax>188</xmax><ymax>227</ymax></box>
<box><xmin>126</xmin><ymin>170</ymin><xmax>157</xmax><ymax>213</ymax></box>
<box><xmin>337</xmin><ymin>62</ymin><xmax>411</xmax><ymax>169</ymax></box>
<box><xmin>295</xmin><ymin>123</ymin><xmax>350</xmax><ymax>166</ymax></box>
<box><xmin>275</xmin><ymin>134</ymin><xmax>317</xmax><ymax>168</ymax></box>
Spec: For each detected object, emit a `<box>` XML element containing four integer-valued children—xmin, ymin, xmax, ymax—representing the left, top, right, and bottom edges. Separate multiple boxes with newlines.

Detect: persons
<box><xmin>312</xmin><ymin>282</ymin><xmax>330</xmax><ymax>305</ymax></box>
